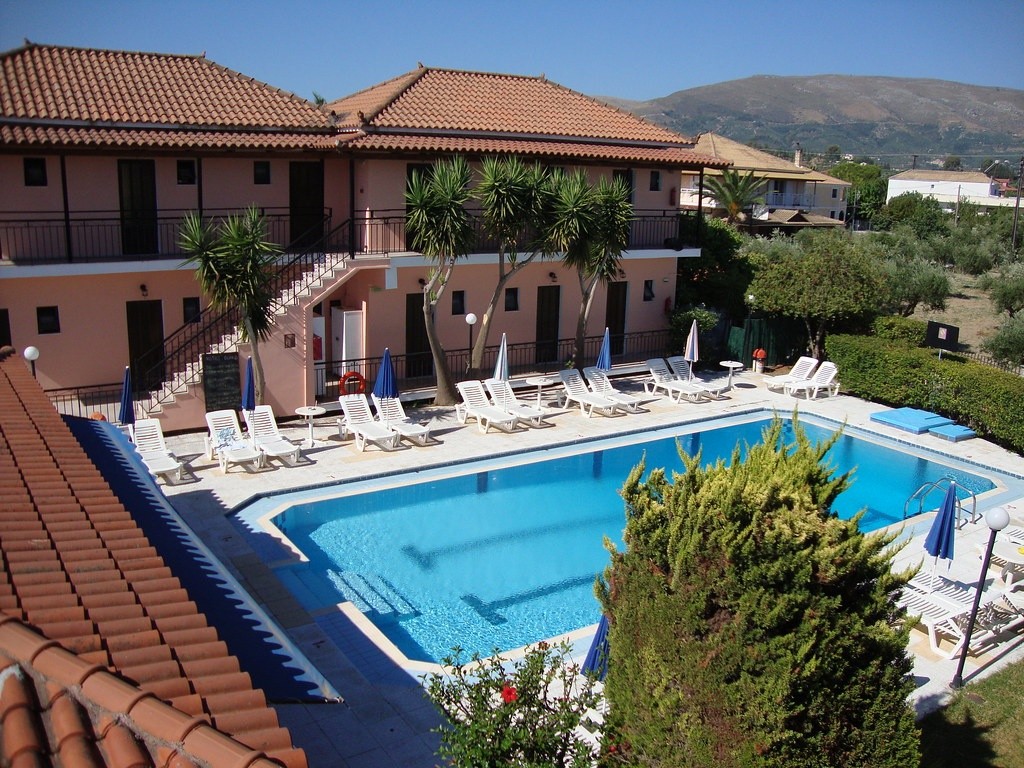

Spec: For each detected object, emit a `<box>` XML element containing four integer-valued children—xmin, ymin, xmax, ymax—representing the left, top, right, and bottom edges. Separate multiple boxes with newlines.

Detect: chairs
<box><xmin>642</xmin><ymin>357</ymin><xmax>704</xmax><ymax>404</ymax></box>
<box><xmin>243</xmin><ymin>404</ymin><xmax>301</xmax><ymax>468</ymax></box>
<box><xmin>485</xmin><ymin>376</ymin><xmax>551</xmax><ymax>428</ymax></box>
<box><xmin>454</xmin><ymin>379</ymin><xmax>519</xmax><ymax>434</ymax></box>
<box><xmin>556</xmin><ymin>368</ymin><xmax>618</xmax><ymax>417</ymax></box>
<box><xmin>203</xmin><ymin>408</ymin><xmax>262</xmax><ymax>474</ymax></box>
<box><xmin>370</xmin><ymin>391</ymin><xmax>429</xmax><ymax>447</ymax></box>
<box><xmin>582</xmin><ymin>366</ymin><xmax>642</xmax><ymax>413</ymax></box>
<box><xmin>336</xmin><ymin>393</ymin><xmax>397</xmax><ymax>452</ymax></box>
<box><xmin>668</xmin><ymin>356</ymin><xmax>726</xmax><ymax>399</ymax></box>
<box><xmin>128</xmin><ymin>418</ymin><xmax>184</xmax><ymax>480</ymax></box>
<box><xmin>762</xmin><ymin>356</ymin><xmax>841</xmax><ymax>403</ymax></box>
<box><xmin>890</xmin><ymin>526</ymin><xmax>1024</xmax><ymax>659</ymax></box>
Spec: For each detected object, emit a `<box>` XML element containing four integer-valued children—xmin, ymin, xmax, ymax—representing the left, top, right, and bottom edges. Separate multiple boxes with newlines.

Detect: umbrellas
<box><xmin>242</xmin><ymin>356</ymin><xmax>255</xmax><ymax>445</ymax></box>
<box><xmin>683</xmin><ymin>319</ymin><xmax>699</xmax><ymax>382</ymax></box>
<box><xmin>118</xmin><ymin>366</ymin><xmax>136</xmax><ymax>425</ymax></box>
<box><xmin>580</xmin><ymin>612</ymin><xmax>611</xmax><ymax>715</ymax></box>
<box><xmin>595</xmin><ymin>327</ymin><xmax>612</xmax><ymax>398</ymax></box>
<box><xmin>372</xmin><ymin>347</ymin><xmax>400</xmax><ymax>428</ymax></box>
<box><xmin>493</xmin><ymin>333</ymin><xmax>510</xmax><ymax>413</ymax></box>
<box><xmin>923</xmin><ymin>481</ymin><xmax>957</xmax><ymax>595</ymax></box>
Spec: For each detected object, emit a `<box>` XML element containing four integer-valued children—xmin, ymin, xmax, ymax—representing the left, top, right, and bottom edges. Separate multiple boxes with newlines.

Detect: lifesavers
<box><xmin>339</xmin><ymin>371</ymin><xmax>365</xmax><ymax>395</ymax></box>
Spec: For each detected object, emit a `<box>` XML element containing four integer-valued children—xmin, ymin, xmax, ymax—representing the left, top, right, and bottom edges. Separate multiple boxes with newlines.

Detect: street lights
<box><xmin>24</xmin><ymin>346</ymin><xmax>40</xmax><ymax>378</ymax></box>
<box><xmin>948</xmin><ymin>507</ymin><xmax>1009</xmax><ymax>690</ymax></box>
<box><xmin>466</xmin><ymin>312</ymin><xmax>477</xmax><ymax>381</ymax></box>
<box><xmin>742</xmin><ymin>294</ymin><xmax>754</xmax><ymax>370</ymax></box>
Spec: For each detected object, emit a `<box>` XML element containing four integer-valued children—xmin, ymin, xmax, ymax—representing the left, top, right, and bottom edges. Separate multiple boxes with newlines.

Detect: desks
<box><xmin>720</xmin><ymin>360</ymin><xmax>744</xmax><ymax>391</ymax></box>
<box><xmin>992</xmin><ymin>542</ymin><xmax>1024</xmax><ymax>583</ymax></box>
<box><xmin>295</xmin><ymin>406</ymin><xmax>327</xmax><ymax>448</ymax></box>
<box><xmin>527</xmin><ymin>376</ymin><xmax>554</xmax><ymax>412</ymax></box>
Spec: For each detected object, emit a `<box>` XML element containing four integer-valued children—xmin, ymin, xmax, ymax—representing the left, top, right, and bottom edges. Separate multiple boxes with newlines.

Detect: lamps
<box><xmin>549</xmin><ymin>272</ymin><xmax>557</xmax><ymax>282</ymax></box>
<box><xmin>617</xmin><ymin>268</ymin><xmax>626</xmax><ymax>278</ymax></box>
<box><xmin>418</xmin><ymin>279</ymin><xmax>427</xmax><ymax>290</ymax></box>
<box><xmin>140</xmin><ymin>284</ymin><xmax>148</xmax><ymax>296</ymax></box>
